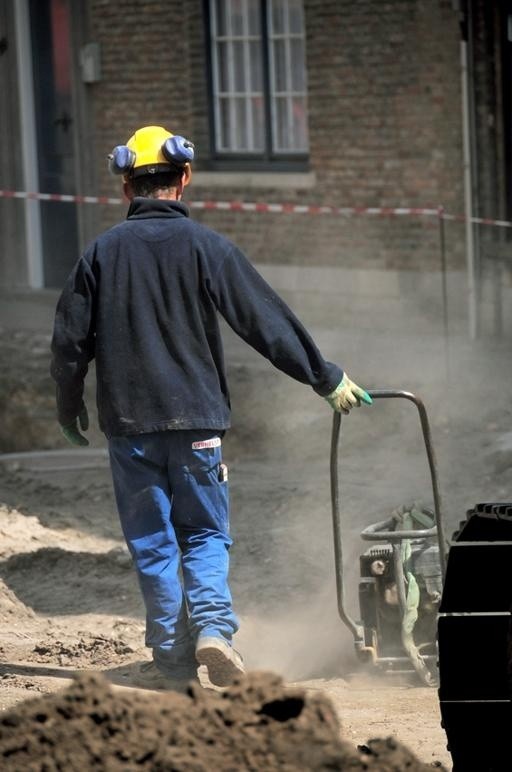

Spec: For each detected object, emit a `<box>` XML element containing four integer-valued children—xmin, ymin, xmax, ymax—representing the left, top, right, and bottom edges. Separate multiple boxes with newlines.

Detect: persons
<box><xmin>48</xmin><ymin>121</ymin><xmax>377</xmax><ymax>690</ymax></box>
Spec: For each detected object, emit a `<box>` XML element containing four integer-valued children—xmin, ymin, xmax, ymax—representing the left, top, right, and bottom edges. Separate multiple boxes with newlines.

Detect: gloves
<box><xmin>325</xmin><ymin>371</ymin><xmax>372</xmax><ymax>415</ymax></box>
<box><xmin>60</xmin><ymin>406</ymin><xmax>88</xmax><ymax>444</ymax></box>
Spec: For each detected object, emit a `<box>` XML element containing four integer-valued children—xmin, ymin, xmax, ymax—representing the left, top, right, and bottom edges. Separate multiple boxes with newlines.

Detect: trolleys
<box><xmin>328</xmin><ymin>386</ymin><xmax>455</xmax><ymax>687</ymax></box>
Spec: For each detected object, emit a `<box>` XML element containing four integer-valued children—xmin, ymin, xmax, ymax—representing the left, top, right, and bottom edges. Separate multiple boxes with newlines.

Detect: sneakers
<box><xmin>195</xmin><ymin>636</ymin><xmax>246</xmax><ymax>686</ymax></box>
<box><xmin>130</xmin><ymin>657</ymin><xmax>202</xmax><ymax>690</ymax></box>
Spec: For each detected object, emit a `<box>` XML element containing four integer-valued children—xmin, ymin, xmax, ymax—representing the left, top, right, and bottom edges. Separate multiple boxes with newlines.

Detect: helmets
<box><xmin>107</xmin><ymin>126</ymin><xmax>196</xmax><ymax>182</ymax></box>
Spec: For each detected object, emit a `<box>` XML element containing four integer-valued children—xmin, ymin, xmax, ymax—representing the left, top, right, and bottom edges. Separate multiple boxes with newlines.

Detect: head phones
<box><xmin>102</xmin><ymin>135</ymin><xmax>198</xmax><ymax>176</ymax></box>
